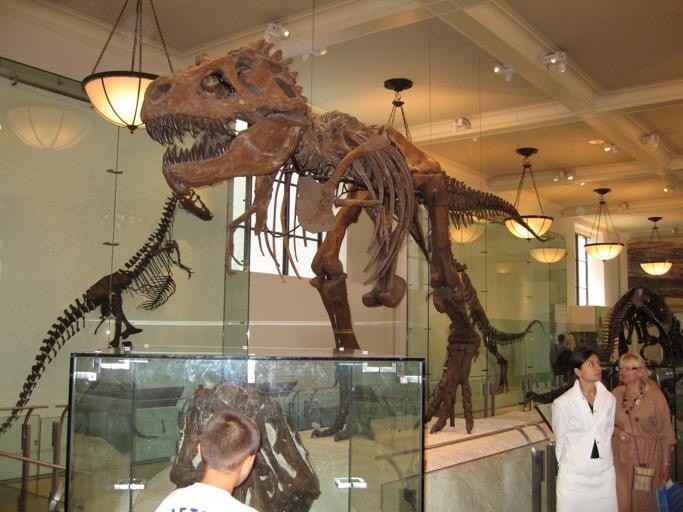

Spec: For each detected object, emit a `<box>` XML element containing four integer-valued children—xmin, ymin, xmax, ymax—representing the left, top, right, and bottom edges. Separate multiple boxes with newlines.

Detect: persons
<box><xmin>150</xmin><ymin>410</ymin><xmax>261</xmax><ymax>512</ymax></box>
<box><xmin>549</xmin><ymin>334</ymin><xmax>573</xmax><ymax>379</ymax></box>
<box><xmin>605</xmin><ymin>352</ymin><xmax>678</xmax><ymax>512</ymax></box>
<box><xmin>552</xmin><ymin>350</ymin><xmax>619</xmax><ymax>512</ymax></box>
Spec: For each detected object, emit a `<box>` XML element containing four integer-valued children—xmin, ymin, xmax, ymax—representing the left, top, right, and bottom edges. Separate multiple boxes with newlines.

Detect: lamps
<box><xmin>503</xmin><ymin>148</ymin><xmax>553</xmax><ymax>241</ymax></box>
<box><xmin>584</xmin><ymin>188</ymin><xmax>624</xmax><ymax>263</ymax></box>
<box><xmin>81</xmin><ymin>0</ymin><xmax>176</xmax><ymax>136</ymax></box>
<box><xmin>639</xmin><ymin>217</ymin><xmax>672</xmax><ymax>277</ymax></box>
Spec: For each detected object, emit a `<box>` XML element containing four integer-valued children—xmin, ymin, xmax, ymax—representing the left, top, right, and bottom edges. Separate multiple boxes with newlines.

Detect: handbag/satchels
<box><xmin>656</xmin><ymin>480</ymin><xmax>682</xmax><ymax>512</ymax></box>
<box><xmin>633</xmin><ymin>467</ymin><xmax>653</xmax><ymax>491</ymax></box>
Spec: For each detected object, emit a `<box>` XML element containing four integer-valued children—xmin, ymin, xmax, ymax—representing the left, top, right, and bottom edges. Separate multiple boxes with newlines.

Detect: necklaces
<box><xmin>621</xmin><ymin>382</ymin><xmax>646</xmax><ymax>414</ymax></box>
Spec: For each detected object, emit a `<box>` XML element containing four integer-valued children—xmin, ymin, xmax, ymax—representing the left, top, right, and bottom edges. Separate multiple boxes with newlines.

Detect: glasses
<box><xmin>616</xmin><ymin>366</ymin><xmax>637</xmax><ymax>370</ymax></box>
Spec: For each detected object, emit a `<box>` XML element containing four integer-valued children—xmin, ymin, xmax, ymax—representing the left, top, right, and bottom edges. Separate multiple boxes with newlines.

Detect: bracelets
<box><xmin>664</xmin><ymin>462</ymin><xmax>671</xmax><ymax>467</ymax></box>
